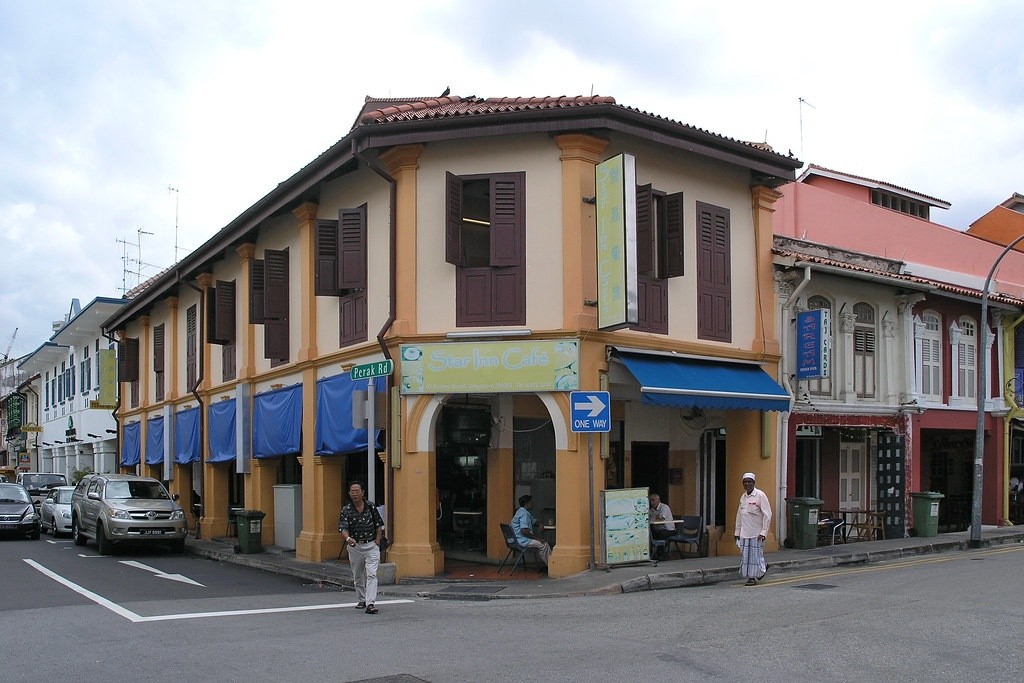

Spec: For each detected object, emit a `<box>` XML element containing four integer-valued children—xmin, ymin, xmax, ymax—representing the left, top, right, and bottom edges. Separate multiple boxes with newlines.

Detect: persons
<box><xmin>192</xmin><ymin>490</ymin><xmax>201</xmax><ymax>516</ymax></box>
<box><xmin>338</xmin><ymin>481</ymin><xmax>384</xmax><ymax>613</ymax></box>
<box><xmin>507</xmin><ymin>494</ymin><xmax>551</xmax><ymax>567</ymax></box>
<box><xmin>650</xmin><ymin>494</ymin><xmax>675</xmax><ymax>540</ymax></box>
<box><xmin>733</xmin><ymin>473</ymin><xmax>773</xmax><ymax>585</ymax></box>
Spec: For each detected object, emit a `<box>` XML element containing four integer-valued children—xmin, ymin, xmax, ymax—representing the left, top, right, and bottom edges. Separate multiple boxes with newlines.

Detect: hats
<box><xmin>742</xmin><ymin>472</ymin><xmax>756</xmax><ymax>481</ymax></box>
<box><xmin>519</xmin><ymin>495</ymin><xmax>532</xmax><ymax>507</ymax></box>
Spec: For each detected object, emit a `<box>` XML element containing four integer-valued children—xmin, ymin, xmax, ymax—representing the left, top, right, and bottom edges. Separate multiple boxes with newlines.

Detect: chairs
<box><xmin>497</xmin><ymin>523</ymin><xmax>543</xmax><ymax>576</ymax></box>
<box><xmin>815</xmin><ymin>506</ymin><xmax>886</xmax><ymax>546</ymax></box>
<box><xmin>664</xmin><ymin>515</ymin><xmax>703</xmax><ymax>560</ymax></box>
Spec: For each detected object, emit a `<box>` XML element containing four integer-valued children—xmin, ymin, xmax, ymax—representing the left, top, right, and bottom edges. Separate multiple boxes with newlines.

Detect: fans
<box><xmin>679</xmin><ymin>406</ymin><xmax>707</xmax><ymax>430</ymax></box>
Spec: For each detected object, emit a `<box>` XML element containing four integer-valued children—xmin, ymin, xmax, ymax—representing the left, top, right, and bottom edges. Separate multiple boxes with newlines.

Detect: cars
<box><xmin>0</xmin><ymin>467</ymin><xmax>16</xmax><ymax>483</ymax></box>
<box><xmin>0</xmin><ymin>483</ymin><xmax>42</xmax><ymax>541</ymax></box>
<box><xmin>39</xmin><ymin>486</ymin><xmax>75</xmax><ymax>538</ymax></box>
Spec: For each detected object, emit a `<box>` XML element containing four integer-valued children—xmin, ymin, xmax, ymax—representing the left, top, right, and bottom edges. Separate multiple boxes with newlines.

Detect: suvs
<box><xmin>70</xmin><ymin>472</ymin><xmax>188</xmax><ymax>557</ymax></box>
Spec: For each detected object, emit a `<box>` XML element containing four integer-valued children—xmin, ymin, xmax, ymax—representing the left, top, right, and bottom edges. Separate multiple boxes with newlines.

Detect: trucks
<box><xmin>16</xmin><ymin>473</ymin><xmax>76</xmax><ymax>516</ymax></box>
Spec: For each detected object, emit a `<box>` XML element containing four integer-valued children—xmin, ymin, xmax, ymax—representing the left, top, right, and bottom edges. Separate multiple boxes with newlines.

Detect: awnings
<box><xmin>611</xmin><ymin>350</ymin><xmax>791</xmax><ymax>413</ymax></box>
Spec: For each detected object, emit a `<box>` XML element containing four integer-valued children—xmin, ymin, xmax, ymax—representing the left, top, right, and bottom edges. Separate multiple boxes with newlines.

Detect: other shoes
<box><xmin>745</xmin><ymin>578</ymin><xmax>757</xmax><ymax>585</ymax></box>
<box><xmin>356</xmin><ymin>602</ymin><xmax>366</xmax><ymax>609</ymax></box>
<box><xmin>365</xmin><ymin>604</ymin><xmax>379</xmax><ymax>612</ymax></box>
<box><xmin>757</xmin><ymin>563</ymin><xmax>770</xmax><ymax>580</ymax></box>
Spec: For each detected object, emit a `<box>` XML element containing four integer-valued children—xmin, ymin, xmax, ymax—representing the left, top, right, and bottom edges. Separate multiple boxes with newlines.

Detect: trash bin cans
<box><xmin>908</xmin><ymin>492</ymin><xmax>945</xmax><ymax>538</ymax></box>
<box><xmin>783</xmin><ymin>497</ymin><xmax>824</xmax><ymax>549</ymax></box>
<box><xmin>233</xmin><ymin>510</ymin><xmax>268</xmax><ymax>553</ymax></box>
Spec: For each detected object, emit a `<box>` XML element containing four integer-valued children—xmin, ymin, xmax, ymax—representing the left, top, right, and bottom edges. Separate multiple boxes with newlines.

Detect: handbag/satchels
<box><xmin>379</xmin><ymin>536</ymin><xmax>388</xmax><ymax>551</ymax></box>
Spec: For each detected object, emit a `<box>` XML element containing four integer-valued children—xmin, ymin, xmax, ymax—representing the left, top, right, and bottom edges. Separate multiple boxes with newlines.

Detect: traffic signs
<box><xmin>569</xmin><ymin>389</ymin><xmax>611</xmax><ymax>434</ymax></box>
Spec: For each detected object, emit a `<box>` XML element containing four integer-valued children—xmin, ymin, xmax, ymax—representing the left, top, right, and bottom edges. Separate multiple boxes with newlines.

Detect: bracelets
<box><xmin>345</xmin><ymin>537</ymin><xmax>351</xmax><ymax>541</ymax></box>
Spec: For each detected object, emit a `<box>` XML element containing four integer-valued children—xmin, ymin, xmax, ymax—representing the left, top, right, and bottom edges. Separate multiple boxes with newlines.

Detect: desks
<box><xmin>649</xmin><ymin>519</ymin><xmax>684</xmax><ymax>561</ymax></box>
<box><xmin>532</xmin><ymin>524</ymin><xmax>556</xmax><ymax>572</ymax></box>
<box><xmin>455</xmin><ymin>512</ymin><xmax>484</xmax><ymax>551</ymax></box>
<box><xmin>815</xmin><ymin>509</ymin><xmax>871</xmax><ymax>544</ymax></box>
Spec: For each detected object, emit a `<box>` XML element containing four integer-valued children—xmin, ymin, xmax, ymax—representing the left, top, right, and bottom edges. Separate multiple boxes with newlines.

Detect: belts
<box><xmin>356</xmin><ymin>539</ymin><xmax>373</xmax><ymax>543</ymax></box>
